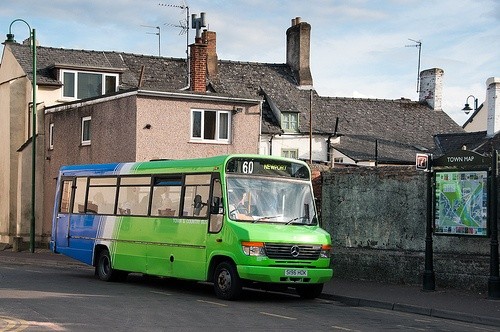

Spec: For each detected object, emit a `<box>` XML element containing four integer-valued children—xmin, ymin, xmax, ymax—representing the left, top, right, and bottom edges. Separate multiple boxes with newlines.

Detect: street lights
<box><xmin>2</xmin><ymin>19</ymin><xmax>37</xmax><ymax>253</ymax></box>
<box><xmin>463</xmin><ymin>95</ymin><xmax>478</xmax><ymax>115</ymax></box>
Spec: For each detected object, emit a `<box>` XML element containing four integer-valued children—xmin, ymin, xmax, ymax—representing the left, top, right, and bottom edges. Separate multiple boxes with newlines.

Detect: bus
<box><xmin>49</xmin><ymin>154</ymin><xmax>333</xmax><ymax>300</ymax></box>
